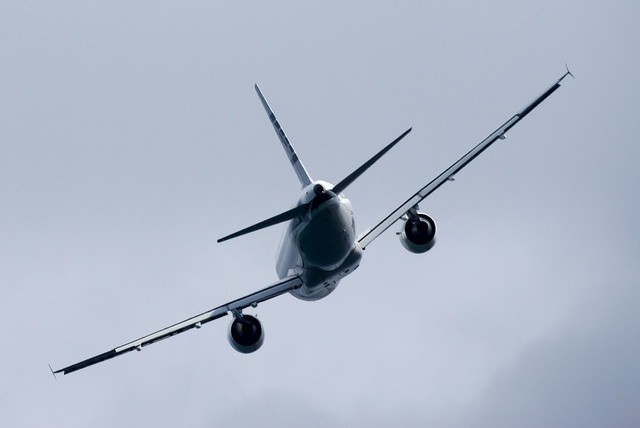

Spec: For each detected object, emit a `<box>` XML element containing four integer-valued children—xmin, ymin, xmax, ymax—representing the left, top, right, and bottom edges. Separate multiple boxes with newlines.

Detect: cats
<box><xmin>48</xmin><ymin>63</ymin><xmax>575</xmax><ymax>380</ymax></box>
